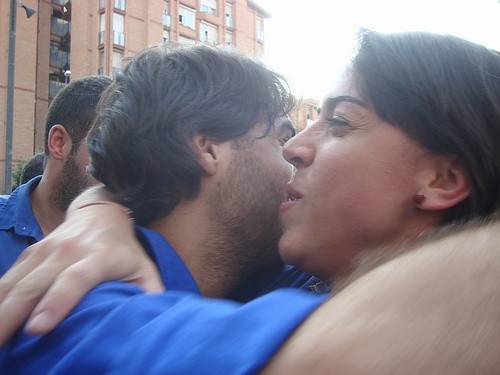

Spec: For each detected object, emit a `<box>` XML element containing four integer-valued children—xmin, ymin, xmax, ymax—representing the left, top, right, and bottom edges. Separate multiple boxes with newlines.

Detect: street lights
<box><xmin>4</xmin><ymin>0</ymin><xmax>38</xmax><ymax>196</ymax></box>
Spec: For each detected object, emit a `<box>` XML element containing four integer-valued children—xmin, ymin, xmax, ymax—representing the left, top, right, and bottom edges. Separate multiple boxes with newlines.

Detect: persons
<box><xmin>1</xmin><ymin>152</ymin><xmax>45</xmax><ymax>208</ymax></box>
<box><xmin>0</xmin><ymin>27</ymin><xmax>500</xmax><ymax>375</ymax></box>
<box><xmin>0</xmin><ymin>74</ymin><xmax>112</xmax><ymax>281</ymax></box>
<box><xmin>0</xmin><ymin>45</ymin><xmax>499</xmax><ymax>375</ymax></box>
